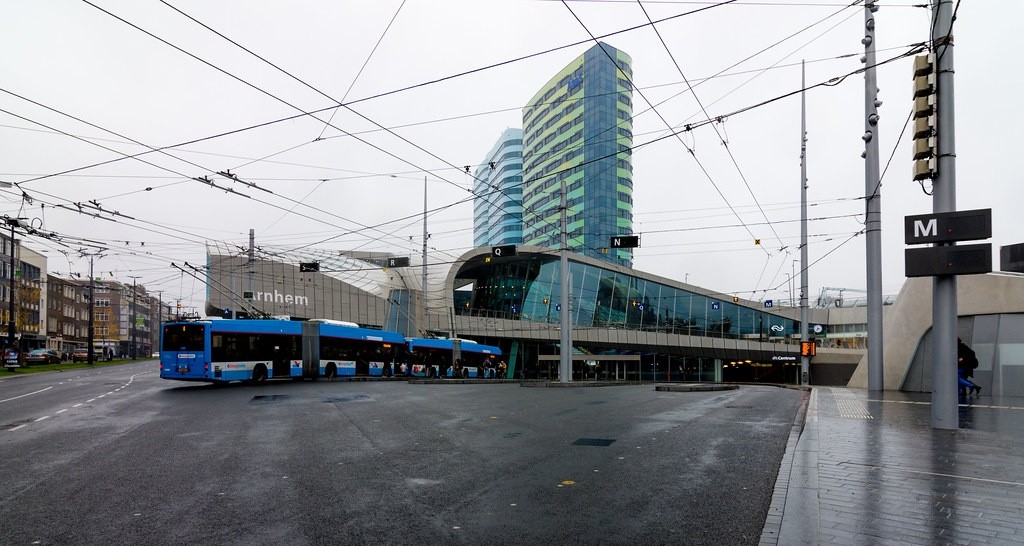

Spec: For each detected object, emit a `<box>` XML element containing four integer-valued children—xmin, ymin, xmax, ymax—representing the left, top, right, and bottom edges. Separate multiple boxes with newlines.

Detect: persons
<box><xmin>106</xmin><ymin>345</ymin><xmax>130</xmax><ymax>364</ymax></box>
<box><xmin>380</xmin><ymin>343</ymin><xmax>697</xmax><ymax>384</ymax></box>
<box><xmin>958</xmin><ymin>335</ymin><xmax>983</xmax><ymax>398</ymax></box>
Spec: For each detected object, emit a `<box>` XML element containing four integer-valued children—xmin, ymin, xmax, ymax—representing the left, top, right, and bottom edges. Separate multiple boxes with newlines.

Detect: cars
<box><xmin>26</xmin><ymin>349</ymin><xmax>62</xmax><ymax>366</ymax></box>
<box><xmin>153</xmin><ymin>352</ymin><xmax>160</xmax><ymax>358</ymax></box>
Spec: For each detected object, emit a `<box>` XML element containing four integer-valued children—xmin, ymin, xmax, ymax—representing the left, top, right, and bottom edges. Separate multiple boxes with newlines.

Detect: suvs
<box><xmin>74</xmin><ymin>349</ymin><xmax>99</xmax><ymax>363</ymax></box>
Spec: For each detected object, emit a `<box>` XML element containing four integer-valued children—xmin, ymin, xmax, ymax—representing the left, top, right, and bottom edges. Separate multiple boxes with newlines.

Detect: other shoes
<box><xmin>958</xmin><ymin>384</ymin><xmax>982</xmax><ymax>396</ymax></box>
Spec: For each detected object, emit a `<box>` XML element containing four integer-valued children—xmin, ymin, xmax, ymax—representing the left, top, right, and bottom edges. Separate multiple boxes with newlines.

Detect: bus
<box><xmin>387</xmin><ymin>298</ymin><xmax>503</xmax><ymax>379</ymax></box>
<box><xmin>159</xmin><ymin>262</ymin><xmax>408</xmax><ymax>386</ymax></box>
